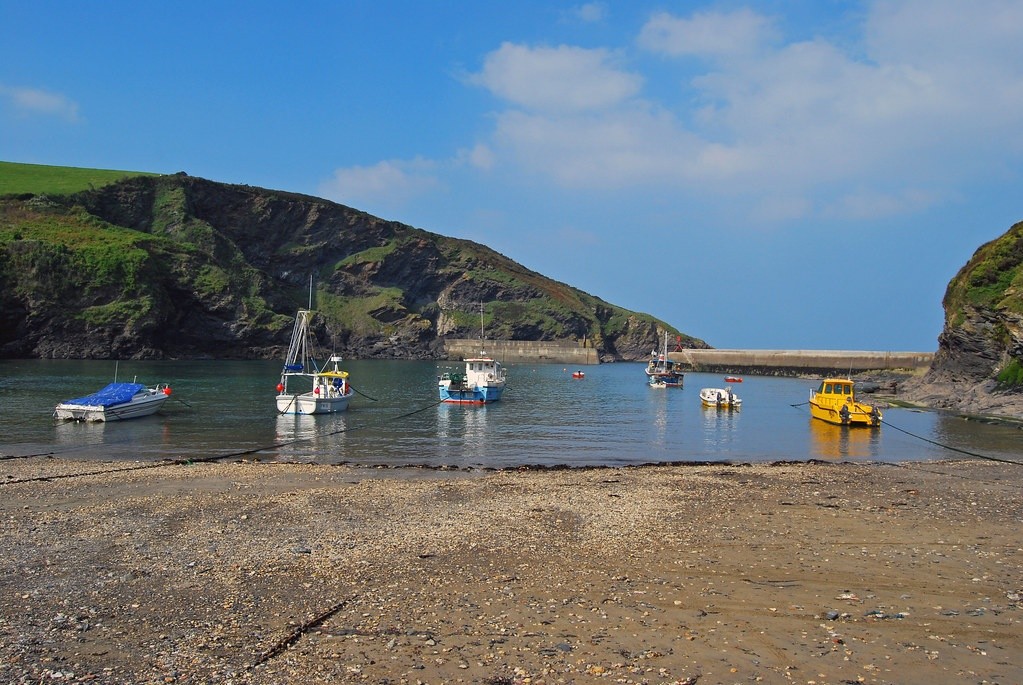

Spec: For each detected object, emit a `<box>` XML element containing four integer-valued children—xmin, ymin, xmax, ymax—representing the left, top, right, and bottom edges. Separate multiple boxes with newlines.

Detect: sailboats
<box><xmin>645</xmin><ymin>330</ymin><xmax>685</xmax><ymax>390</ymax></box>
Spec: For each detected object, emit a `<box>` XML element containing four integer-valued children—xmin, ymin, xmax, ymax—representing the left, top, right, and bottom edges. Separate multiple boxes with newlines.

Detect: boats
<box><xmin>699</xmin><ymin>387</ymin><xmax>742</xmax><ymax>407</ymax></box>
<box><xmin>274</xmin><ymin>273</ymin><xmax>355</xmax><ymax>416</ymax></box>
<box><xmin>436</xmin><ymin>299</ymin><xmax>508</xmax><ymax>405</ymax></box>
<box><xmin>808</xmin><ymin>377</ymin><xmax>883</xmax><ymax>427</ymax></box>
<box><xmin>572</xmin><ymin>369</ymin><xmax>585</xmax><ymax>379</ymax></box>
<box><xmin>723</xmin><ymin>375</ymin><xmax>743</xmax><ymax>382</ymax></box>
<box><xmin>52</xmin><ymin>361</ymin><xmax>173</xmax><ymax>423</ymax></box>
